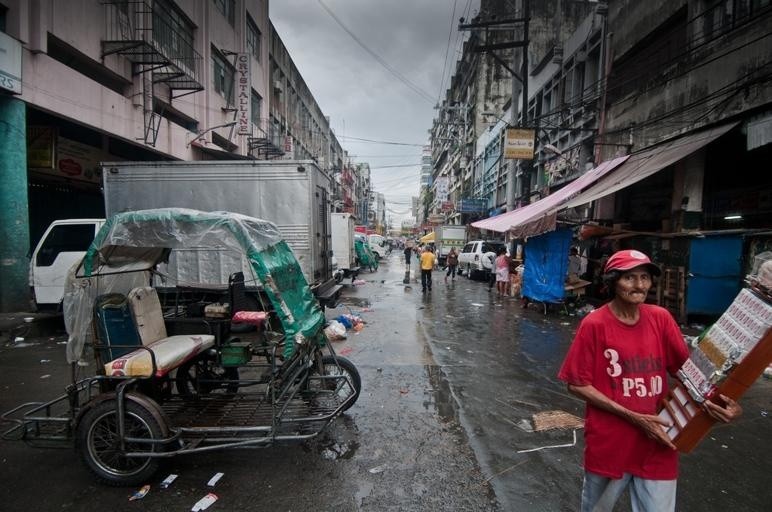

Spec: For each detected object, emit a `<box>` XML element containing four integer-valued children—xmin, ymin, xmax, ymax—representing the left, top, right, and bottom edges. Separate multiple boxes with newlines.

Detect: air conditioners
<box><xmin>273</xmin><ymin>80</ymin><xmax>283</xmax><ymax>93</ymax></box>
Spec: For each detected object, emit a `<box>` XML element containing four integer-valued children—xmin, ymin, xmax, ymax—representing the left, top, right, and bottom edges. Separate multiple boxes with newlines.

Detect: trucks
<box><xmin>433</xmin><ymin>224</ymin><xmax>470</xmax><ymax>268</ymax></box>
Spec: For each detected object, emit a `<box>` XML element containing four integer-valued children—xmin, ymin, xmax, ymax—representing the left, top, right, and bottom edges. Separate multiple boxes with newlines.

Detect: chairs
<box><xmin>657</xmin><ymin>267</ymin><xmax>685</xmax><ymax>317</ymax></box>
<box><xmin>228</xmin><ymin>270</ymin><xmax>270</xmax><ymax>331</ymax></box>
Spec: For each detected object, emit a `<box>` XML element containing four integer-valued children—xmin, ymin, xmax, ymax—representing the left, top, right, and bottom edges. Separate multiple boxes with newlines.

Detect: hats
<box><xmin>603</xmin><ymin>249</ymin><xmax>662</xmax><ymax>277</ymax></box>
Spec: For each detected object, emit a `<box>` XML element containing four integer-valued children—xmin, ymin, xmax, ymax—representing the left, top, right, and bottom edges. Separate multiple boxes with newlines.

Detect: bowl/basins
<box><xmin>24</xmin><ymin>318</ymin><xmax>34</xmax><ymax>323</ymax></box>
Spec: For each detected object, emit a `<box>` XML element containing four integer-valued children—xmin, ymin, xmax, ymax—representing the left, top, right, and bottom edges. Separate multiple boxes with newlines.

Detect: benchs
<box><xmin>93</xmin><ymin>286</ymin><xmax>216</xmax><ymax>378</ymax></box>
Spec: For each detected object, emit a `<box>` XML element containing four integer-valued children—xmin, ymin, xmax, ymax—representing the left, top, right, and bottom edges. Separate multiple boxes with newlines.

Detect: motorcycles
<box><xmin>175</xmin><ymin>300</ymin><xmax>361</xmax><ymax>414</ymax></box>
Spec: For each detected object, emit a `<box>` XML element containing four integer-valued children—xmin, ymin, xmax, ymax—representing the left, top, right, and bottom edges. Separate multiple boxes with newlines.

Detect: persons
<box><xmin>445</xmin><ymin>246</ymin><xmax>459</xmax><ymax>281</ymax></box>
<box><xmin>565</xmin><ymin>247</ymin><xmax>581</xmax><ymax>277</ymax></box>
<box><xmin>555</xmin><ymin>249</ymin><xmax>744</xmax><ymax>511</ymax></box>
<box><xmin>496</xmin><ymin>249</ymin><xmax>504</xmax><ymax>294</ymax></box>
<box><xmin>419</xmin><ymin>245</ymin><xmax>436</xmax><ymax>292</ymax></box>
<box><xmin>414</xmin><ymin>242</ymin><xmax>441</xmax><ymax>271</ymax></box>
<box><xmin>494</xmin><ymin>247</ymin><xmax>513</xmax><ymax>296</ymax></box>
<box><xmin>487</xmin><ymin>247</ymin><xmax>500</xmax><ymax>292</ymax></box>
<box><xmin>404</xmin><ymin>245</ymin><xmax>413</xmax><ymax>271</ymax></box>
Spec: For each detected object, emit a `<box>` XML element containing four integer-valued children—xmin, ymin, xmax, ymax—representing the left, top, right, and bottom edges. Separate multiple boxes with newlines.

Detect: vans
<box><xmin>457</xmin><ymin>240</ymin><xmax>507</xmax><ymax>281</ymax></box>
